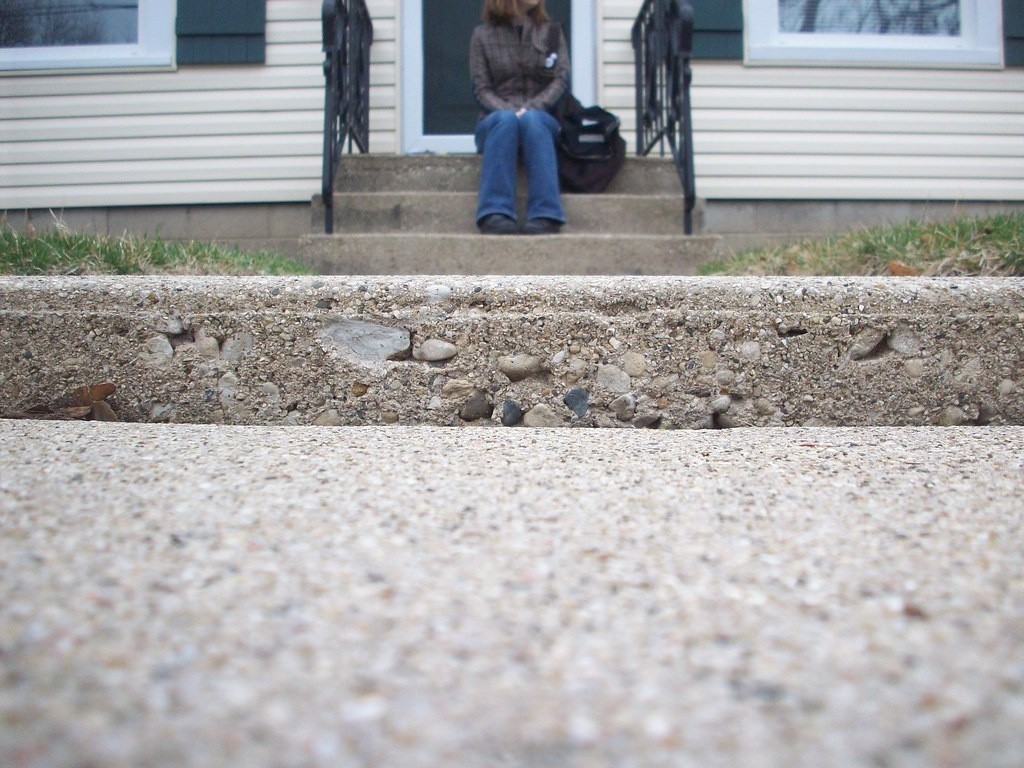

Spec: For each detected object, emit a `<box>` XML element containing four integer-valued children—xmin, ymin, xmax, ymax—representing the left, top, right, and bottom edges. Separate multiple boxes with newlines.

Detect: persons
<box><xmin>469</xmin><ymin>0</ymin><xmax>569</xmax><ymax>234</ymax></box>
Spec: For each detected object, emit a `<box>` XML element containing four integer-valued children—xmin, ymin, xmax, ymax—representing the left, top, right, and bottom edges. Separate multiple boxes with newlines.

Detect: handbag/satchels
<box><xmin>556</xmin><ymin>92</ymin><xmax>626</xmax><ymax>194</ymax></box>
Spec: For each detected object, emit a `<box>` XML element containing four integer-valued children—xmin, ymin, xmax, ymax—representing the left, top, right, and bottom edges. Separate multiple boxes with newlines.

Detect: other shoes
<box><xmin>480</xmin><ymin>213</ymin><xmax>519</xmax><ymax>234</ymax></box>
<box><xmin>520</xmin><ymin>217</ymin><xmax>559</xmax><ymax>234</ymax></box>
